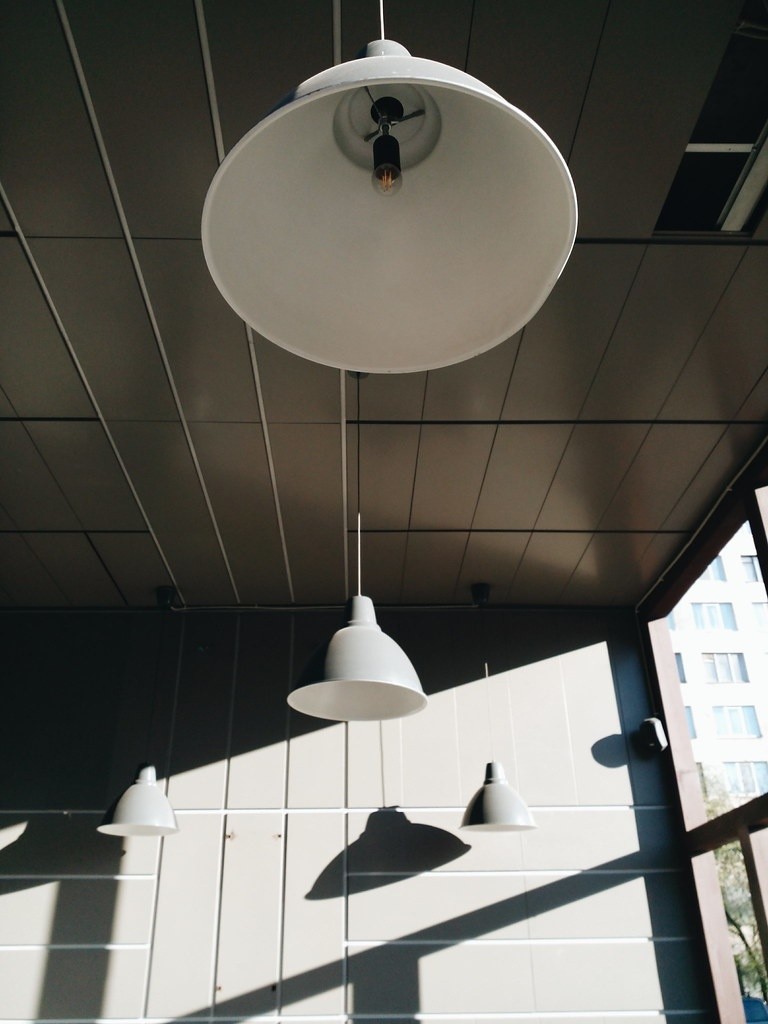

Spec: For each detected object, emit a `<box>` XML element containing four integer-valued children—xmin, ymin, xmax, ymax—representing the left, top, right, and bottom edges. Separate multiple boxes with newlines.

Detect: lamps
<box><xmin>199</xmin><ymin>0</ymin><xmax>580</xmax><ymax>375</ymax></box>
<box><xmin>458</xmin><ymin>581</ymin><xmax>536</xmax><ymax>832</ymax></box>
<box><xmin>286</xmin><ymin>370</ymin><xmax>429</xmax><ymax>723</ymax></box>
<box><xmin>95</xmin><ymin>584</ymin><xmax>194</xmax><ymax>837</ymax></box>
